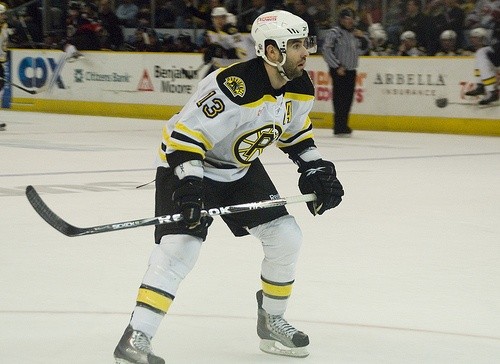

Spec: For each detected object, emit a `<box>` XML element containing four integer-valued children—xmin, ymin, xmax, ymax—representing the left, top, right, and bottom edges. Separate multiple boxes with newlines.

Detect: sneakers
<box><xmin>256</xmin><ymin>289</ymin><xmax>311</xmax><ymax>357</ymax></box>
<box><xmin>0</xmin><ymin>122</ymin><xmax>6</xmax><ymax>131</ymax></box>
<box><xmin>464</xmin><ymin>84</ymin><xmax>487</xmax><ymax>99</ymax></box>
<box><xmin>113</xmin><ymin>311</ymin><xmax>166</xmax><ymax>364</ymax></box>
<box><xmin>478</xmin><ymin>91</ymin><xmax>500</xmax><ymax>108</ymax></box>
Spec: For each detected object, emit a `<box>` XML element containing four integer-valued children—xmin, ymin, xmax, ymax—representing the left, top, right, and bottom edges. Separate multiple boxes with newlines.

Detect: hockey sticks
<box><xmin>24</xmin><ymin>185</ymin><xmax>318</xmax><ymax>239</ymax></box>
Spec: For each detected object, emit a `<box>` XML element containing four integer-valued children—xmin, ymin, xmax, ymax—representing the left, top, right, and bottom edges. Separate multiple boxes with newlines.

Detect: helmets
<box><xmin>440</xmin><ymin>30</ymin><xmax>457</xmax><ymax>41</ymax></box>
<box><xmin>368</xmin><ymin>24</ymin><xmax>388</xmax><ymax>42</ymax></box>
<box><xmin>0</xmin><ymin>3</ymin><xmax>8</xmax><ymax>14</ymax></box>
<box><xmin>252</xmin><ymin>10</ymin><xmax>310</xmax><ymax>56</ymax></box>
<box><xmin>210</xmin><ymin>6</ymin><xmax>229</xmax><ymax>17</ymax></box>
<box><xmin>400</xmin><ymin>31</ymin><xmax>417</xmax><ymax>41</ymax></box>
<box><xmin>225</xmin><ymin>11</ymin><xmax>238</xmax><ymax>27</ymax></box>
<box><xmin>470</xmin><ymin>28</ymin><xmax>486</xmax><ymax>38</ymax></box>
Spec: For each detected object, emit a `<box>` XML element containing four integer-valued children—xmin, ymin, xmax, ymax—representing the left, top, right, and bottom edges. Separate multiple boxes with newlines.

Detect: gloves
<box><xmin>171</xmin><ymin>181</ymin><xmax>213</xmax><ymax>236</ymax></box>
<box><xmin>299</xmin><ymin>161</ymin><xmax>344</xmax><ymax>216</ymax></box>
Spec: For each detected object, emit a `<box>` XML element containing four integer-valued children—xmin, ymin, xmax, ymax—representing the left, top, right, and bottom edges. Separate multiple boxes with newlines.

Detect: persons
<box><xmin>323</xmin><ymin>7</ymin><xmax>361</xmax><ymax>134</ymax></box>
<box><xmin>113</xmin><ymin>10</ymin><xmax>345</xmax><ymax>364</ymax></box>
<box><xmin>0</xmin><ymin>4</ymin><xmax>9</xmax><ymax>127</ymax></box>
<box><xmin>0</xmin><ymin>0</ymin><xmax>500</xmax><ymax>56</ymax></box>
<box><xmin>464</xmin><ymin>28</ymin><xmax>500</xmax><ymax>105</ymax></box>
<box><xmin>201</xmin><ymin>7</ymin><xmax>248</xmax><ymax>77</ymax></box>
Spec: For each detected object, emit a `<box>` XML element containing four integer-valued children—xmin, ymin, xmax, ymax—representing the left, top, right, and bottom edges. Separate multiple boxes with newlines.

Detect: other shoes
<box><xmin>334</xmin><ymin>126</ymin><xmax>352</xmax><ymax>136</ymax></box>
